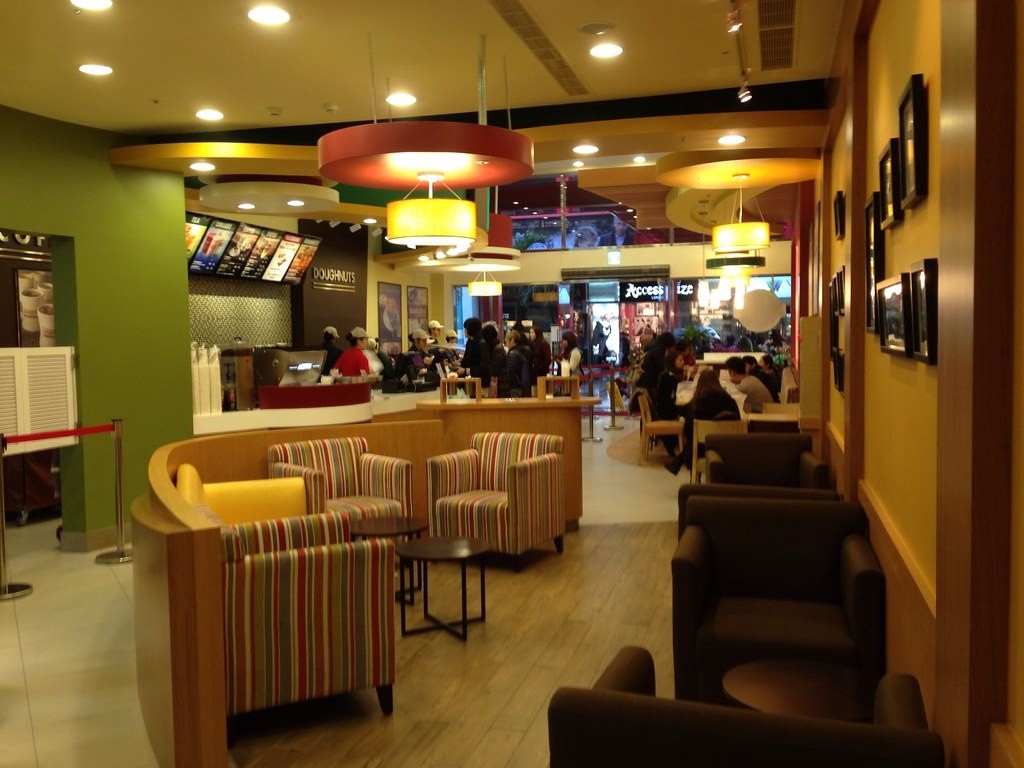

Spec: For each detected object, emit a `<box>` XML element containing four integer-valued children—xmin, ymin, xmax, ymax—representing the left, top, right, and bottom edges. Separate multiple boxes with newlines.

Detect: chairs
<box><xmin>177</xmin><ymin>377</ymin><xmax>949</xmax><ymax>768</ymax></box>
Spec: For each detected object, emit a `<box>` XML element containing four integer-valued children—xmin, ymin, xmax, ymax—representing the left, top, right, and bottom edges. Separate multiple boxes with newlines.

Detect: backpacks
<box><xmin>510</xmin><ymin>349</ymin><xmax>537</xmax><ymax>397</ymax></box>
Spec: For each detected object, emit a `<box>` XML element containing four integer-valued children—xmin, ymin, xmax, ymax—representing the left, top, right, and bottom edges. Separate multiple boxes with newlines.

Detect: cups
<box><xmin>202</xmin><ymin>236</ymin><xmax>213</xmax><ymax>253</ymax></box>
<box><xmin>36</xmin><ymin>283</ymin><xmax>53</xmax><ymax>302</ymax></box>
<box><xmin>191</xmin><ymin>350</ymin><xmax>201</xmax><ymax>414</ymax></box>
<box><xmin>210</xmin><ymin>348</ymin><xmax>222</xmax><ymax>414</ymax></box>
<box><xmin>214</xmin><ymin>240</ymin><xmax>224</xmax><ymax>255</ymax></box>
<box><xmin>34</xmin><ymin>271</ymin><xmax>52</xmax><ymax>284</ymax></box>
<box><xmin>199</xmin><ymin>349</ymin><xmax>211</xmax><ymax>414</ymax></box>
<box><xmin>206</xmin><ymin>239</ymin><xmax>220</xmax><ymax>256</ymax></box>
<box><xmin>37</xmin><ymin>304</ymin><xmax>56</xmax><ymax>347</ymax></box>
<box><xmin>233</xmin><ymin>246</ymin><xmax>238</xmax><ymax>253</ymax></box>
<box><xmin>21</xmin><ymin>289</ymin><xmax>42</xmax><ymax>332</ymax></box>
<box><xmin>19</xmin><ymin>273</ymin><xmax>33</xmax><ymax>301</ymax></box>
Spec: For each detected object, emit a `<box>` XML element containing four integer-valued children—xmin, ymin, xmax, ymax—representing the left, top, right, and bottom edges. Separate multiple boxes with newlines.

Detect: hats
<box><xmin>351</xmin><ymin>327</ymin><xmax>372</xmax><ymax>338</ymax></box>
<box><xmin>412</xmin><ymin>329</ymin><xmax>431</xmax><ymax>340</ymax></box>
<box><xmin>368</xmin><ymin>338</ymin><xmax>377</xmax><ymax>350</ymax></box>
<box><xmin>428</xmin><ymin>320</ymin><xmax>444</xmax><ymax>329</ymax></box>
<box><xmin>324</xmin><ymin>326</ymin><xmax>339</xmax><ymax>338</ymax></box>
<box><xmin>446</xmin><ymin>329</ymin><xmax>459</xmax><ymax>337</ymax></box>
<box><xmin>512</xmin><ymin>323</ymin><xmax>525</xmax><ymax>331</ymax></box>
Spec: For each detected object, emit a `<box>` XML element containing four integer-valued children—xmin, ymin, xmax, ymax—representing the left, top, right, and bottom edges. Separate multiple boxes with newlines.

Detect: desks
<box><xmin>396</xmin><ymin>536</ymin><xmax>488</xmax><ymax>642</ymax></box>
<box><xmin>350</xmin><ymin>514</ymin><xmax>429</xmax><ymax>611</ymax></box>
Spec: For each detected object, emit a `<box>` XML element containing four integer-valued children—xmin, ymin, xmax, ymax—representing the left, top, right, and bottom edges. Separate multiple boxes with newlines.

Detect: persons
<box><xmin>395</xmin><ymin>317</ymin><xmax>583</xmax><ymax>398</ymax></box>
<box><xmin>573</xmin><ymin>217</ymin><xmax>633</xmax><ymax>247</ymax></box>
<box><xmin>334</xmin><ymin>326</ymin><xmax>394</xmax><ymax>387</ymax></box>
<box><xmin>305</xmin><ymin>326</ymin><xmax>343</xmax><ymax>376</ymax></box>
<box><xmin>593</xmin><ymin>314</ymin><xmax>788</xmax><ymax>475</ymax></box>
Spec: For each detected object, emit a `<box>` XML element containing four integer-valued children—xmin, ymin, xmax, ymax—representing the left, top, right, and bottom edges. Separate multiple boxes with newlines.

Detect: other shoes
<box><xmin>664</xmin><ymin>461</ymin><xmax>681</xmax><ymax>475</ymax></box>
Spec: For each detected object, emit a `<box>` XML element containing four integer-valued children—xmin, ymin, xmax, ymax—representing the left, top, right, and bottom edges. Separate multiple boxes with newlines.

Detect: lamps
<box><xmin>533</xmin><ymin>284</ymin><xmax>558</xmax><ymax>301</ymax></box>
<box><xmin>727</xmin><ymin>12</ymin><xmax>742</xmax><ymax>33</ymax></box>
<box><xmin>738</xmin><ymin>81</ymin><xmax>751</xmax><ymax>102</ymax></box>
<box><xmin>469</xmin><ymin>269</ymin><xmax>502</xmax><ymax>296</ymax></box>
<box><xmin>713</xmin><ymin>177</ymin><xmax>771</xmax><ymax>250</ymax></box>
<box><xmin>387</xmin><ymin>152</ymin><xmax>476</xmax><ymax>246</ymax></box>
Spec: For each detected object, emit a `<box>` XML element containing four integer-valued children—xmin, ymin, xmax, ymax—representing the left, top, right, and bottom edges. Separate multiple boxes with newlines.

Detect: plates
<box><xmin>229</xmin><ymin>250</ymin><xmax>240</xmax><ymax>257</ymax></box>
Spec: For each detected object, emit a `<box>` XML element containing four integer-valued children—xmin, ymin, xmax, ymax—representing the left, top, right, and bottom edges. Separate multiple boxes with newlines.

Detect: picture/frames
<box><xmin>828</xmin><ymin>73</ymin><xmax>936</xmax><ymax>395</ymax></box>
